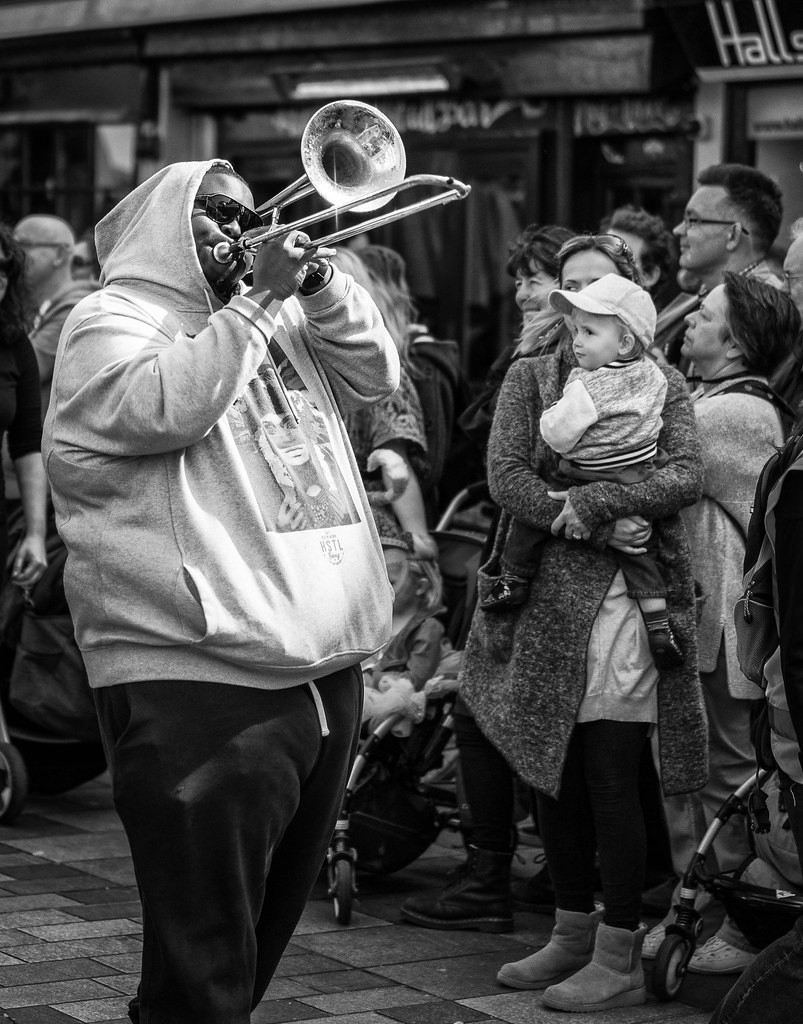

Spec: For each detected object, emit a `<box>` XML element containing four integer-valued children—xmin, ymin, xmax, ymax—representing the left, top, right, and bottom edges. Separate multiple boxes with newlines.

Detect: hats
<box><xmin>548</xmin><ymin>273</ymin><xmax>657</xmax><ymax>350</ymax></box>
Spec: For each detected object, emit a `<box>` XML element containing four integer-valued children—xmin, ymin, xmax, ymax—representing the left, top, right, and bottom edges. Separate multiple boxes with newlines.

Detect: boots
<box><xmin>497</xmin><ymin>900</ymin><xmax>606</xmax><ymax>990</ymax></box>
<box><xmin>511</xmin><ymin>850</ymin><xmax>556</xmax><ymax>915</ymax></box>
<box><xmin>540</xmin><ymin>921</ymin><xmax>646</xmax><ymax>1013</ymax></box>
<box><xmin>399</xmin><ymin>844</ymin><xmax>514</xmax><ymax>933</ymax></box>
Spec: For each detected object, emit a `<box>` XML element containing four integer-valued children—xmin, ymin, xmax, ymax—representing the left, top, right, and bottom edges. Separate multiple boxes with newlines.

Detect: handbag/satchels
<box><xmin>8</xmin><ymin>611</ymin><xmax>103</xmax><ymax>743</ymax></box>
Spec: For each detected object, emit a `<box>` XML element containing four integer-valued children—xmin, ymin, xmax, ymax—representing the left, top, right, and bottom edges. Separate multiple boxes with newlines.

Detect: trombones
<box><xmin>212</xmin><ymin>100</ymin><xmax>472</xmax><ymax>264</ymax></box>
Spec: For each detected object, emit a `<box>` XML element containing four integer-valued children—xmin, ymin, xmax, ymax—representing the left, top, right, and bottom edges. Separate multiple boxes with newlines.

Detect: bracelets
<box><xmin>303</xmin><ymin>264</ymin><xmax>332</xmax><ymax>288</ymax></box>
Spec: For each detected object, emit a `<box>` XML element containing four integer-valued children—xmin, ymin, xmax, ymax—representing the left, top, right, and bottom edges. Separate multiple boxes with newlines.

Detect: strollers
<box><xmin>641</xmin><ymin>770</ymin><xmax>803</xmax><ymax>1001</ymax></box>
<box><xmin>325</xmin><ymin>480</ymin><xmax>500</xmax><ymax>926</ymax></box>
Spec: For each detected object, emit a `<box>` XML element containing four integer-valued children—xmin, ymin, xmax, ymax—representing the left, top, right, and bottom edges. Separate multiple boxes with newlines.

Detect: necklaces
<box><xmin>738</xmin><ymin>257</ymin><xmax>769</xmax><ymax>277</ymax></box>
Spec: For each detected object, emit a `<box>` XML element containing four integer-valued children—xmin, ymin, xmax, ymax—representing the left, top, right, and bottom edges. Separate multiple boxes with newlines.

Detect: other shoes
<box><xmin>649</xmin><ymin>628</ymin><xmax>683</xmax><ymax>666</ymax></box>
<box><xmin>480</xmin><ymin>577</ymin><xmax>527</xmax><ymax>611</ymax></box>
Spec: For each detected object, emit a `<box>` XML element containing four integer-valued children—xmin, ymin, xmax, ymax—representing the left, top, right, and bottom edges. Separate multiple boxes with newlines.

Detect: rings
<box><xmin>573</xmin><ymin>534</ymin><xmax>583</xmax><ymax>539</ymax></box>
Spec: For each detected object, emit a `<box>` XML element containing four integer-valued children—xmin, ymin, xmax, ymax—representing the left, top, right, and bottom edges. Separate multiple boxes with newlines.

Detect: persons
<box><xmin>478</xmin><ymin>274</ymin><xmax>683</xmax><ymax>667</ymax></box>
<box><xmin>458</xmin><ymin>236</ymin><xmax>711</xmax><ymax>1012</ymax></box>
<box><xmin>2</xmin><ymin>160</ymin><xmax>803</xmax><ymax>1024</ymax></box>
<box><xmin>41</xmin><ymin>158</ymin><xmax>402</xmax><ymax>1024</ymax></box>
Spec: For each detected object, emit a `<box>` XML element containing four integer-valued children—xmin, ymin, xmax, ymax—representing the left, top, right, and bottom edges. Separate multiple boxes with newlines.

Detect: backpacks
<box><xmin>688</xmin><ymin>380</ymin><xmax>802</xmax><ymax>690</ymax></box>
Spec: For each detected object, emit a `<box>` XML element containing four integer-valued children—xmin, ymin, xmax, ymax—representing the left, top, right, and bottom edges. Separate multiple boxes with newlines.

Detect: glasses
<box><xmin>685</xmin><ymin>216</ymin><xmax>749</xmax><ymax>235</ymax></box>
<box><xmin>562</xmin><ymin>234</ymin><xmax>631</xmax><ymax>265</ymax></box>
<box><xmin>194</xmin><ymin>192</ymin><xmax>265</xmax><ymax>235</ymax></box>
<box><xmin>15</xmin><ymin>239</ymin><xmax>69</xmax><ymax>252</ymax></box>
<box><xmin>782</xmin><ymin>273</ymin><xmax>800</xmax><ymax>295</ymax></box>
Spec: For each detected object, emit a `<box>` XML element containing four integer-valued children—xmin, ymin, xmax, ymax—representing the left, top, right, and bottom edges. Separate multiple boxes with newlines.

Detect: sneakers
<box><xmin>686</xmin><ymin>936</ymin><xmax>757</xmax><ymax>974</ymax></box>
<box><xmin>641</xmin><ymin>923</ymin><xmax>667</xmax><ymax>960</ymax></box>
